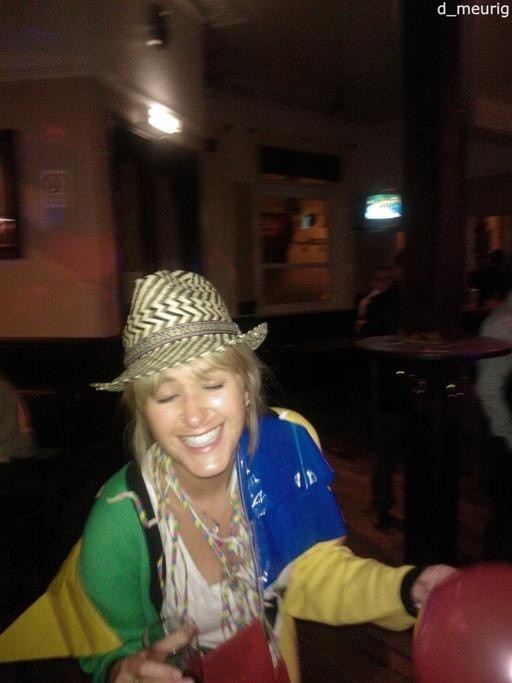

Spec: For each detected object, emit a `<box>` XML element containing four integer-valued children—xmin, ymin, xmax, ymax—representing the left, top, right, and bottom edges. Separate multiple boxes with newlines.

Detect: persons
<box><xmin>72</xmin><ymin>267</ymin><xmax>460</xmax><ymax>681</ymax></box>
<box><xmin>1</xmin><ymin>374</ymin><xmax>40</xmax><ymax>549</ymax></box>
<box><xmin>347</xmin><ymin>243</ymin><xmax>510</xmax><ymax>567</ymax></box>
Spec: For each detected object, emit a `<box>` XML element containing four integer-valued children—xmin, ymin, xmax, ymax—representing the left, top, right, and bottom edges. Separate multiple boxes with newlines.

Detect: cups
<box><xmin>141</xmin><ymin>616</ymin><xmax>205</xmax><ymax>683</ymax></box>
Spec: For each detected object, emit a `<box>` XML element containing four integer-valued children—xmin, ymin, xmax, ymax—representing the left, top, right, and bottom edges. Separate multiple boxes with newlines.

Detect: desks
<box><xmin>356</xmin><ymin>334</ymin><xmax>511</xmax><ymax>571</ymax></box>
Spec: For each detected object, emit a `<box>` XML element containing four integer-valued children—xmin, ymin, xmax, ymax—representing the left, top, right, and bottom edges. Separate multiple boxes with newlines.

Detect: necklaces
<box><xmin>169</xmin><ymin>469</ymin><xmax>233</xmax><ymax>538</ymax></box>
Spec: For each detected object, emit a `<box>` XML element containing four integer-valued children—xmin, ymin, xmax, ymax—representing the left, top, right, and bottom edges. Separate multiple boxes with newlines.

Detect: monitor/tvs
<box><xmin>355</xmin><ymin>187</ymin><xmax>406</xmax><ymax>228</ymax></box>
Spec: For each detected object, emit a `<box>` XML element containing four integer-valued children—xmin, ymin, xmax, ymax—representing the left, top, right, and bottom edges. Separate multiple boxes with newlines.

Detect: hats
<box><xmin>91</xmin><ymin>270</ymin><xmax>270</xmax><ymax>392</ymax></box>
